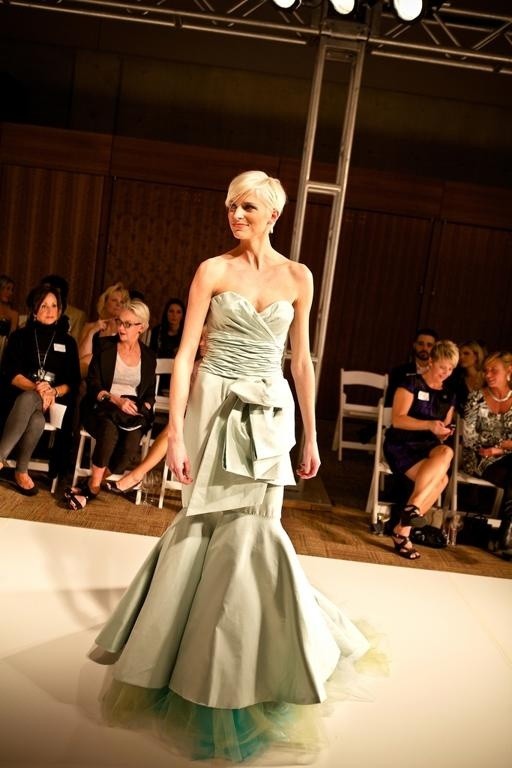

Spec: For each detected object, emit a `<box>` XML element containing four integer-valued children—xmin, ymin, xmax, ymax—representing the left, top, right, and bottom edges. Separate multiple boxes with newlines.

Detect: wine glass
<box><xmin>141</xmin><ymin>470</ymin><xmax>162</xmax><ymax>507</ymax></box>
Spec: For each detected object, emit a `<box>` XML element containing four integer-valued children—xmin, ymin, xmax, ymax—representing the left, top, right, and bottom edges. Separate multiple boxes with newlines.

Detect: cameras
<box><xmin>34</xmin><ymin>368</ymin><xmax>55</xmax><ymax>382</ymax></box>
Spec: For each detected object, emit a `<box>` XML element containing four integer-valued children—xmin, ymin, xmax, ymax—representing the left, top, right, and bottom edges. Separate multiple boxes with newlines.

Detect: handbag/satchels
<box><xmin>456</xmin><ymin>513</ymin><xmax>492</xmax><ymax>549</ymax></box>
<box><xmin>412</xmin><ymin>524</ymin><xmax>446</xmax><ymax>548</ymax></box>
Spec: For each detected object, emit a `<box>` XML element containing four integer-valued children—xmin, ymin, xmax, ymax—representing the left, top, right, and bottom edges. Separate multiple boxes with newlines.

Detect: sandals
<box><xmin>391</xmin><ymin>531</ymin><xmax>421</xmax><ymax>558</ymax></box>
<box><xmin>399</xmin><ymin>502</ymin><xmax>427</xmax><ymax>529</ymax></box>
<box><xmin>67</xmin><ymin>489</ymin><xmax>98</xmax><ymax>510</ymax></box>
<box><xmin>64</xmin><ymin>482</ymin><xmax>86</xmax><ymax>498</ymax></box>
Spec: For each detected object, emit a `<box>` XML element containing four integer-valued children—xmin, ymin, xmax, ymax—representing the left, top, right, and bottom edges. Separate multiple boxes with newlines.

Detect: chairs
<box><xmin>365</xmin><ymin>397</ymin><xmax>460</xmax><ymax>547</ymax></box>
<box><xmin>2</xmin><ymin>347</ymin><xmax>206</xmax><ymax>512</ymax></box>
<box><xmin>330</xmin><ymin>365</ymin><xmax>390</xmax><ymax>464</ymax></box>
<box><xmin>448</xmin><ymin>414</ymin><xmax>512</xmax><ymax>543</ymax></box>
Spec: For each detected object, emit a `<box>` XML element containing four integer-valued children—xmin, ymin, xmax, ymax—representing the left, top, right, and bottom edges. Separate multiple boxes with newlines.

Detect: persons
<box><xmin>0</xmin><ymin>275</ymin><xmax>207</xmax><ymax>511</ymax></box>
<box><xmin>384</xmin><ymin>328</ymin><xmax>438</xmax><ymax>407</ymax></box>
<box><xmin>87</xmin><ymin>170</ymin><xmax>369</xmax><ymax>764</ymax></box>
<box><xmin>459</xmin><ymin>351</ymin><xmax>512</xmax><ymax>554</ymax></box>
<box><xmin>453</xmin><ymin>340</ymin><xmax>484</xmax><ymax>402</ymax></box>
<box><xmin>383</xmin><ymin>340</ymin><xmax>459</xmax><ymax>560</ymax></box>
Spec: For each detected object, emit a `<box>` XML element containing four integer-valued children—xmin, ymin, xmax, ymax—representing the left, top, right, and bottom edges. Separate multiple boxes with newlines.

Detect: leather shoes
<box><xmin>107</xmin><ymin>478</ymin><xmax>136</xmax><ymax>496</ymax></box>
<box><xmin>12</xmin><ymin>471</ymin><xmax>38</xmax><ymax>496</ymax></box>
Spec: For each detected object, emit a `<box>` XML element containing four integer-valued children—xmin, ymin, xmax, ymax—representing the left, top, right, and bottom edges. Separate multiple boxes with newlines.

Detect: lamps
<box><xmin>274</xmin><ymin>0</ymin><xmax>448</xmax><ymax>27</ymax></box>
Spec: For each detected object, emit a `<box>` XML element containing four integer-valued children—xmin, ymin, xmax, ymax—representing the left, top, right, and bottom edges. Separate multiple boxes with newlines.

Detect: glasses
<box><xmin>115</xmin><ymin>319</ymin><xmax>143</xmax><ymax>328</ymax></box>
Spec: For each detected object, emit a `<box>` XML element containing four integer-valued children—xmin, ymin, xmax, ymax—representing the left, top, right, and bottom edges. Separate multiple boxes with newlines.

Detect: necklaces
<box><xmin>486</xmin><ymin>387</ymin><xmax>512</xmax><ymax>402</ymax></box>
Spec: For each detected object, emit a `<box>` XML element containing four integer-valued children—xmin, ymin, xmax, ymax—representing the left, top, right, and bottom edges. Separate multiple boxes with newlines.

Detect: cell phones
<box><xmin>445</xmin><ymin>424</ymin><xmax>456</xmax><ymax>428</ymax></box>
